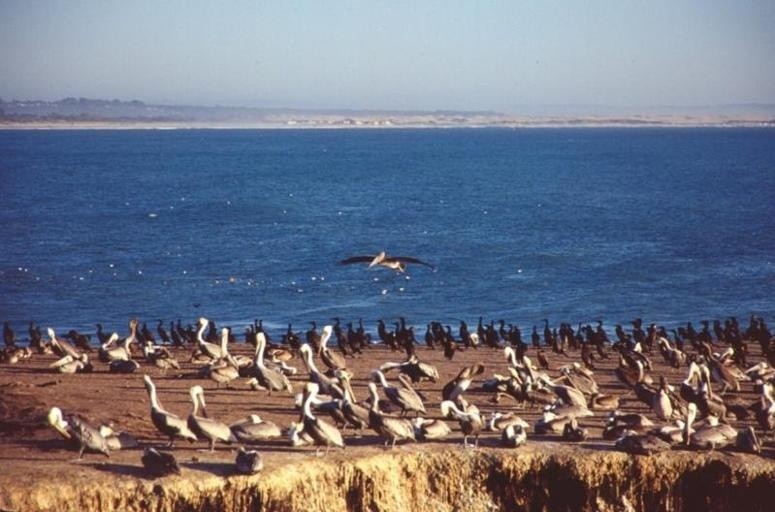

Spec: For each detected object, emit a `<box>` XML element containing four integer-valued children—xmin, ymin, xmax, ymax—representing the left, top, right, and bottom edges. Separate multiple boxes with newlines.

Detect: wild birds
<box><xmin>2</xmin><ymin>310</ymin><xmax>774</xmax><ymax>458</ymax></box>
<box><xmin>235</xmin><ymin>446</ymin><xmax>263</xmax><ymax>475</ymax></box>
<box><xmin>340</xmin><ymin>250</ymin><xmax>435</xmax><ymax>275</ymax></box>
<box><xmin>142</xmin><ymin>446</ymin><xmax>182</xmax><ymax>477</ymax></box>
